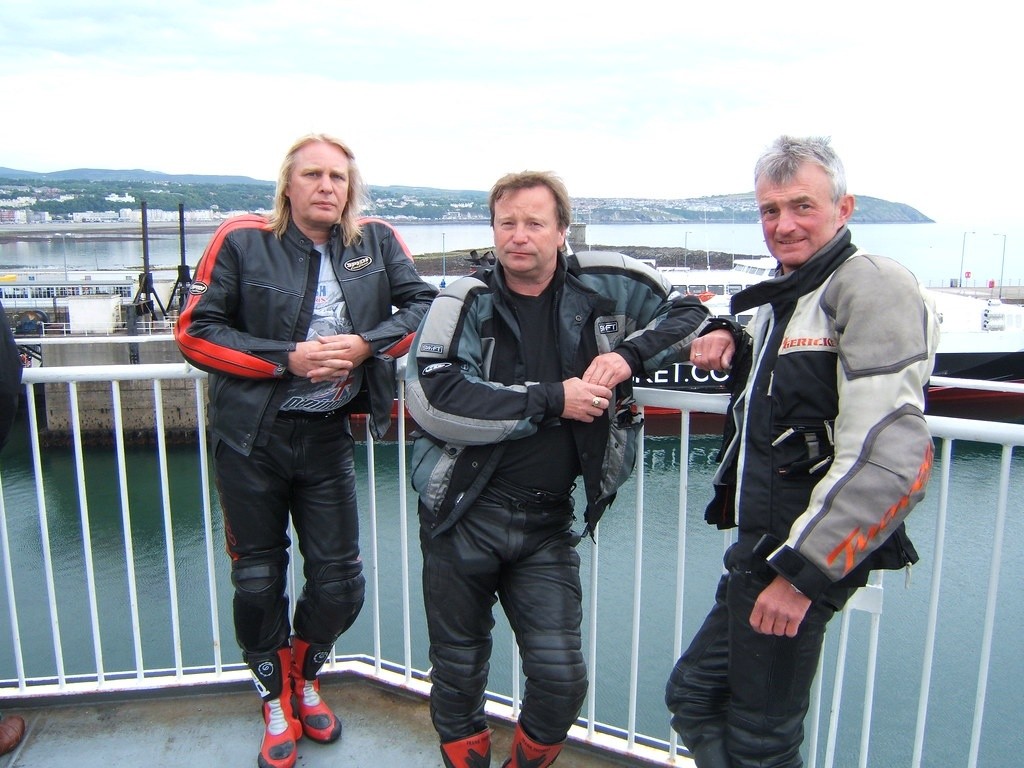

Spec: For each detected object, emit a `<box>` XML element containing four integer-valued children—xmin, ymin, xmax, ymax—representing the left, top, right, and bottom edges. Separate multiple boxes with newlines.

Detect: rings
<box><xmin>593</xmin><ymin>397</ymin><xmax>601</xmax><ymax>407</ymax></box>
<box><xmin>695</xmin><ymin>353</ymin><xmax>702</xmax><ymax>356</ymax></box>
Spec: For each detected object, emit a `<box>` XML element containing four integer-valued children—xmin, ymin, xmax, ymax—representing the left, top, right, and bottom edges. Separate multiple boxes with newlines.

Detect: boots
<box><xmin>242</xmin><ymin>640</ymin><xmax>302</xmax><ymax>768</ymax></box>
<box><xmin>290</xmin><ymin>635</ymin><xmax>342</xmax><ymax>743</ymax></box>
<box><xmin>440</xmin><ymin>725</ymin><xmax>491</xmax><ymax>768</ymax></box>
<box><xmin>502</xmin><ymin>713</ymin><xmax>568</xmax><ymax>768</ymax></box>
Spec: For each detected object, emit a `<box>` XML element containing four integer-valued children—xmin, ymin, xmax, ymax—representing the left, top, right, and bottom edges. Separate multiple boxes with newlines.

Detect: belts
<box><xmin>277</xmin><ymin>399</ymin><xmax>353</xmax><ymax>421</ymax></box>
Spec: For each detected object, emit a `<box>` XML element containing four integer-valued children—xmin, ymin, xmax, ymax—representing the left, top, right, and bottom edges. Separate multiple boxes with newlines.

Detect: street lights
<box><xmin>55</xmin><ymin>232</ymin><xmax>72</xmax><ymax>279</ymax></box>
<box><xmin>960</xmin><ymin>230</ymin><xmax>976</xmax><ymax>292</ymax></box>
<box><xmin>685</xmin><ymin>232</ymin><xmax>692</xmax><ymax>268</ymax></box>
<box><xmin>994</xmin><ymin>232</ymin><xmax>1007</xmax><ymax>299</ymax></box>
<box><xmin>442</xmin><ymin>231</ymin><xmax>448</xmax><ymax>282</ymax></box>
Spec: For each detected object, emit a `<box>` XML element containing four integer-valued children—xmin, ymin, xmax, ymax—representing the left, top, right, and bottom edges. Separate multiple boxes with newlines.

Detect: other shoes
<box><xmin>0</xmin><ymin>716</ymin><xmax>25</xmax><ymax>756</ymax></box>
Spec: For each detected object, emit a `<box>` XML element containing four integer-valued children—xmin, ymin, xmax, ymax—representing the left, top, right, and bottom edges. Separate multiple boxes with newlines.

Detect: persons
<box><xmin>405</xmin><ymin>169</ymin><xmax>716</xmax><ymax>768</ymax></box>
<box><xmin>664</xmin><ymin>134</ymin><xmax>939</xmax><ymax>768</ymax></box>
<box><xmin>173</xmin><ymin>133</ymin><xmax>440</xmax><ymax>768</ymax></box>
<box><xmin>0</xmin><ymin>304</ymin><xmax>26</xmax><ymax>757</ymax></box>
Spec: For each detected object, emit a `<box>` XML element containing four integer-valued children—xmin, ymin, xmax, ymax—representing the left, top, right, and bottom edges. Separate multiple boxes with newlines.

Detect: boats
<box><xmin>2</xmin><ymin>254</ymin><xmax>1024</xmax><ymax>442</ymax></box>
